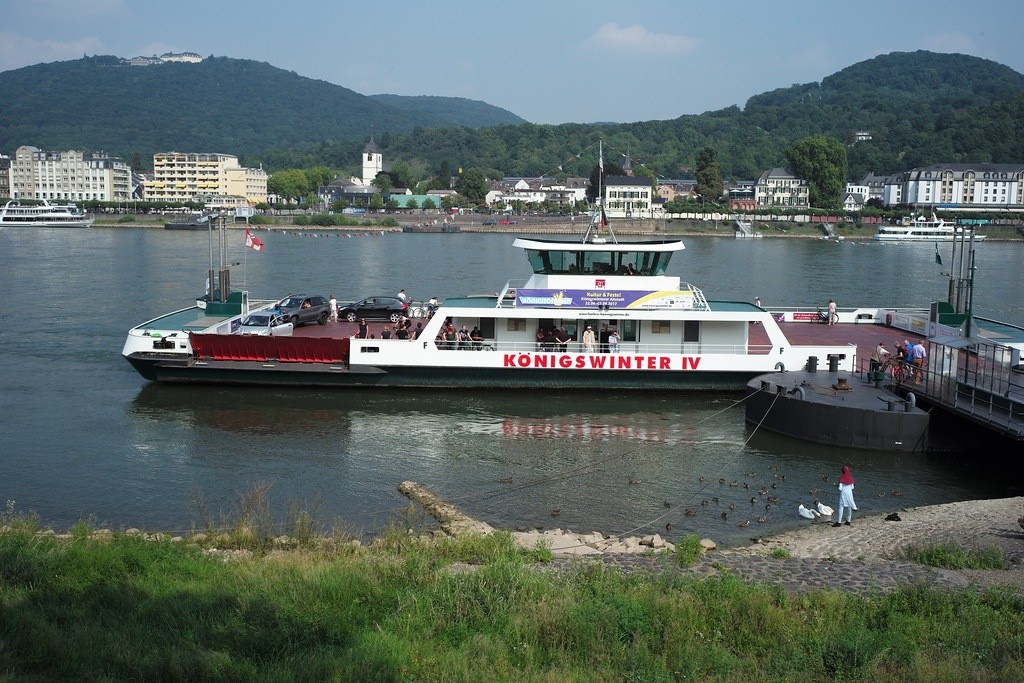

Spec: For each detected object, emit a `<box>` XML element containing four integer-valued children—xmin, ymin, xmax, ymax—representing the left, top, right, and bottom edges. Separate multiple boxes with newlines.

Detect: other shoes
<box><xmin>845</xmin><ymin>520</ymin><xmax>850</xmax><ymax>525</ymax></box>
<box><xmin>832</xmin><ymin>522</ymin><xmax>841</xmax><ymax>527</ymax></box>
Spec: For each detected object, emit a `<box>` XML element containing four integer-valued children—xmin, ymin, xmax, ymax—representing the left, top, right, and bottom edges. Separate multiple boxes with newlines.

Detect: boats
<box><xmin>733</xmin><ymin>212</ymin><xmax>763</xmax><ymax>239</ymax></box>
<box><xmin>121</xmin><ymin>139</ymin><xmax>1024</xmax><ymax>394</ymax></box>
<box><xmin>873</xmin><ymin>201</ymin><xmax>987</xmax><ymax>242</ymax></box>
<box><xmin>0</xmin><ymin>199</ymin><xmax>95</xmax><ymax>228</ymax></box>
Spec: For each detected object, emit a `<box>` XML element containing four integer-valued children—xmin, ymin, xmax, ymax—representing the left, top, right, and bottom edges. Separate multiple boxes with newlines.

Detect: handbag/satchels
<box><xmin>885</xmin><ymin>512</ymin><xmax>901</xmax><ymax>521</ymax></box>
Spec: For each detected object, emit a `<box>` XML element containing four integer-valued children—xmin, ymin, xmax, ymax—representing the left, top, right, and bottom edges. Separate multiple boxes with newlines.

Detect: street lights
<box><xmin>99</xmin><ymin>205</ymin><xmax>100</xmax><ymax>215</ymax></box>
<box><xmin>83</xmin><ymin>203</ymin><xmax>85</xmax><ymax>214</ymax></box>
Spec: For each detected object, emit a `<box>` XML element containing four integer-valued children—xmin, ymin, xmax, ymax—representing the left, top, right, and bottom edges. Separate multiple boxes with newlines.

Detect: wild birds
<box><xmin>666</xmin><ymin>522</ymin><xmax>672</xmax><ymax>530</ymax></box>
<box><xmin>853</xmin><ymin>505</ymin><xmax>859</xmax><ymax>511</ymax></box>
<box><xmin>664</xmin><ymin>501</ymin><xmax>670</xmax><ymax>506</ymax></box>
<box><xmin>686</xmin><ymin>509</ymin><xmax>698</xmax><ymax>516</ymax></box>
<box><xmin>628</xmin><ymin>478</ymin><xmax>642</xmax><ymax>484</ymax></box>
<box><xmin>498</xmin><ymin>477</ymin><xmax>513</xmax><ymax>485</ymax></box>
<box><xmin>891</xmin><ymin>490</ymin><xmax>901</xmax><ymax>496</ymax></box>
<box><xmin>696</xmin><ymin>471</ymin><xmax>828</xmax><ymax>543</ymax></box>
<box><xmin>878</xmin><ymin>492</ymin><xmax>887</xmax><ymax>497</ymax></box>
<box><xmin>551</xmin><ymin>508</ymin><xmax>561</xmax><ymax>516</ymax></box>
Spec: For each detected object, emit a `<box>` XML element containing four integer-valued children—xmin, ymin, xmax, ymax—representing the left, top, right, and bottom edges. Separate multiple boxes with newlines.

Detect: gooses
<box><xmin>818</xmin><ymin>502</ymin><xmax>835</xmax><ymax>516</ymax></box>
<box><xmin>799</xmin><ymin>504</ymin><xmax>821</xmax><ymax>519</ymax></box>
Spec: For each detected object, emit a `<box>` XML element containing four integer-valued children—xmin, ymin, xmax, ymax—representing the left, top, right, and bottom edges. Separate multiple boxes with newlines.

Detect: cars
<box><xmin>509</xmin><ymin>220</ymin><xmax>518</xmax><ymax>224</ymax></box>
<box><xmin>502</xmin><ymin>209</ymin><xmax>589</xmax><ymax>220</ymax></box>
<box><xmin>896</xmin><ymin>220</ymin><xmax>902</xmax><ymax>226</ymax></box>
<box><xmin>890</xmin><ymin>218</ymin><xmax>896</xmax><ymax>224</ymax></box>
<box><xmin>499</xmin><ymin>221</ymin><xmax>511</xmax><ymax>225</ymax></box>
<box><xmin>82</xmin><ymin>208</ymin><xmax>161</xmax><ymax>215</ymax></box>
<box><xmin>482</xmin><ymin>219</ymin><xmax>497</xmax><ymax>225</ymax></box>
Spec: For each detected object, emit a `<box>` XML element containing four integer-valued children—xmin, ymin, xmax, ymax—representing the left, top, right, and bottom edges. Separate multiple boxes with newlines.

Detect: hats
<box><xmin>587</xmin><ymin>326</ymin><xmax>592</xmax><ymax>329</ymax></box>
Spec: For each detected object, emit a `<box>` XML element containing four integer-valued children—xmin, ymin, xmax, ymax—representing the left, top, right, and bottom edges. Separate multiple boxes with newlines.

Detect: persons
<box><xmin>623</xmin><ymin>262</ymin><xmax>635</xmax><ymax>275</ymax></box>
<box><xmin>280</xmin><ymin>309</ymin><xmax>289</xmax><ymax>323</ymax></box>
<box><xmin>828</xmin><ymin>298</ymin><xmax>836</xmax><ymax>326</ymax></box>
<box><xmin>507</xmin><ymin>291</ymin><xmax>514</xmax><ymax>297</ymax></box>
<box><xmin>753</xmin><ymin>296</ymin><xmax>760</xmax><ymax>324</ymax></box>
<box><xmin>583</xmin><ymin>326</ymin><xmax>595</xmax><ymax>353</ymax></box>
<box><xmin>608</xmin><ymin>331</ymin><xmax>620</xmax><ymax>353</ymax></box>
<box><xmin>304</xmin><ymin>300</ymin><xmax>311</xmax><ymax>308</ymax></box>
<box><xmin>536</xmin><ymin>326</ymin><xmax>571</xmax><ymax>353</ymax></box>
<box><xmin>356</xmin><ymin>289</ymin><xmax>485</xmax><ymax>351</ymax></box>
<box><xmin>831</xmin><ymin>466</ymin><xmax>858</xmax><ymax>527</ymax></box>
<box><xmin>326</xmin><ymin>294</ymin><xmax>338</xmax><ymax>322</ymax></box>
<box><xmin>870</xmin><ymin>339</ymin><xmax>927</xmax><ymax>384</ymax></box>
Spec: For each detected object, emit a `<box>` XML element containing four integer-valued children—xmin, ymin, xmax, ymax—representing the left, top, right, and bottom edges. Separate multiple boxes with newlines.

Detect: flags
<box><xmin>602</xmin><ymin>206</ymin><xmax>608</xmax><ymax>230</ymax></box>
<box><xmin>935</xmin><ymin>242</ymin><xmax>943</xmax><ymax>265</ymax></box>
<box><xmin>599</xmin><ymin>140</ymin><xmax>603</xmax><ymax>172</ymax></box>
<box><xmin>245</xmin><ymin>228</ymin><xmax>263</xmax><ymax>252</ymax></box>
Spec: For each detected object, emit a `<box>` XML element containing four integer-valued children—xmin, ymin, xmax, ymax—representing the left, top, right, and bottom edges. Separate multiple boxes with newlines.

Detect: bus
<box><xmin>902</xmin><ymin>217</ymin><xmax>910</xmax><ymax>227</ymax></box>
<box><xmin>161</xmin><ymin>208</ymin><xmax>189</xmax><ymax>215</ymax></box>
